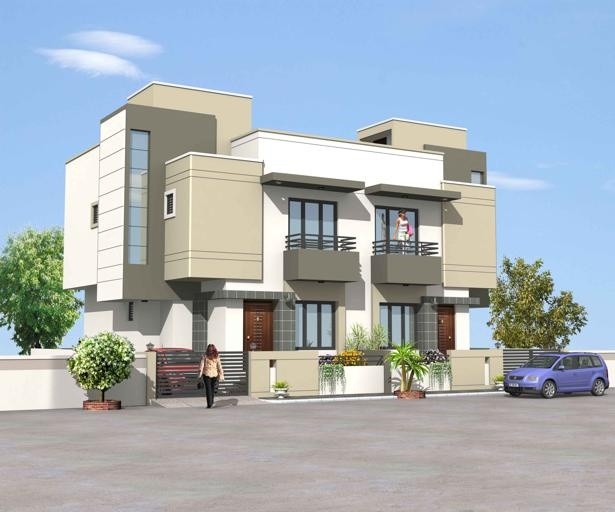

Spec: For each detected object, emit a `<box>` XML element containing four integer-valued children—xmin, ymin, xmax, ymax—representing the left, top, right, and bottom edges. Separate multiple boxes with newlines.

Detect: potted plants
<box><xmin>66</xmin><ymin>328</ymin><xmax>136</xmax><ymax>411</ymax></box>
<box><xmin>491</xmin><ymin>373</ymin><xmax>506</xmax><ymax>391</ymax></box>
<box><xmin>383</xmin><ymin>341</ymin><xmax>433</xmax><ymax>398</ymax></box>
<box><xmin>271</xmin><ymin>379</ymin><xmax>292</xmax><ymax>398</ymax></box>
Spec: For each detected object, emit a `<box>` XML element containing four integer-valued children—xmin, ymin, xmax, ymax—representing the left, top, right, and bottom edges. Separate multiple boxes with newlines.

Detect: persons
<box><xmin>196</xmin><ymin>344</ymin><xmax>225</xmax><ymax>409</ymax></box>
<box><xmin>393</xmin><ymin>207</ymin><xmax>411</xmax><ymax>252</ymax></box>
